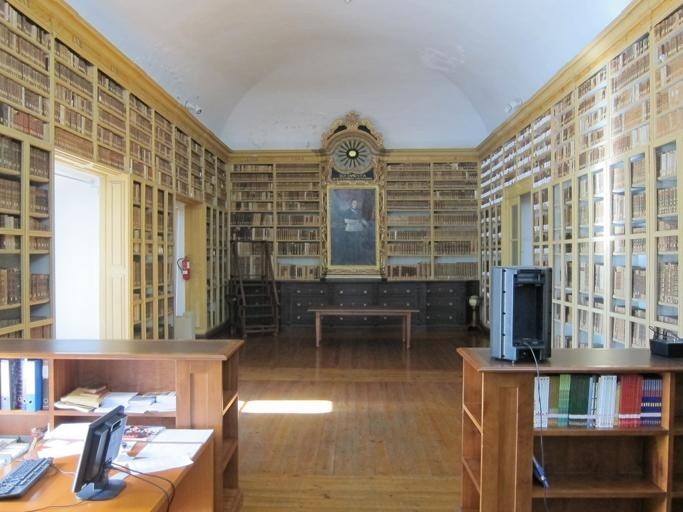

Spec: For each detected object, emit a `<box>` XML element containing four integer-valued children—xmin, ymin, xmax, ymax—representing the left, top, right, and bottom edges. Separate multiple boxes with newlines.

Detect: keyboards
<box><xmin>0</xmin><ymin>457</ymin><xmax>53</xmax><ymax>499</ymax></box>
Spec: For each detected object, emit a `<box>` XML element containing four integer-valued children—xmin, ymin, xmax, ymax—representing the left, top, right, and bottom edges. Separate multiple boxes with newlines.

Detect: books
<box><xmin>0</xmin><ymin>359</ymin><xmax>49</xmax><ymax>409</ymax></box>
<box><xmin>229</xmin><ymin>164</ymin><xmax>321</xmax><ymax>280</ymax></box>
<box><xmin>533</xmin><ymin>373</ymin><xmax>664</xmax><ymax>429</ymax></box>
<box><xmin>0</xmin><ymin>1</ymin><xmax>229</xmax><ymax>341</ymax></box>
<box><xmin>479</xmin><ymin>4</ymin><xmax>682</xmax><ymax>348</ymax></box>
<box><xmin>53</xmin><ymin>386</ymin><xmax>178</xmax><ymax>417</ymax></box>
<box><xmin>387</xmin><ymin>163</ymin><xmax>479</xmax><ymax>279</ymax></box>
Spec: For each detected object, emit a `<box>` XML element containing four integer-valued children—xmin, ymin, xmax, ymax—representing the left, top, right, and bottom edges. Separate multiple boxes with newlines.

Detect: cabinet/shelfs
<box><xmin>478</xmin><ymin>2</ymin><xmax>680</xmax><ymax>347</ymax></box>
<box><xmin>455</xmin><ymin>345</ymin><xmax>680</xmax><ymax>510</ymax></box>
<box><xmin>1</xmin><ymin>1</ymin><xmax>233</xmax><ymax>342</ymax></box>
<box><xmin>2</xmin><ymin>336</ymin><xmax>245</xmax><ymax>511</ymax></box>
<box><xmin>232</xmin><ymin>109</ymin><xmax>479</xmax><ymax>328</ymax></box>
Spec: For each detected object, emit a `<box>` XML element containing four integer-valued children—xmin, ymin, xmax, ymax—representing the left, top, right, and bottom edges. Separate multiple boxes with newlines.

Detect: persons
<box><xmin>342</xmin><ymin>198</ymin><xmax>368</xmax><ymax>264</ymax></box>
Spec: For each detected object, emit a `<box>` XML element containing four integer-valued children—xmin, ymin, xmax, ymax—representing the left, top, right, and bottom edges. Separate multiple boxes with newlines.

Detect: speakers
<box><xmin>489</xmin><ymin>266</ymin><xmax>552</xmax><ymax>361</ymax></box>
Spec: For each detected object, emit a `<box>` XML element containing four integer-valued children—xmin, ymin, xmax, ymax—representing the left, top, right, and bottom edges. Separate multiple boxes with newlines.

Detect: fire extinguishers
<box><xmin>177</xmin><ymin>255</ymin><xmax>190</xmax><ymax>280</ymax></box>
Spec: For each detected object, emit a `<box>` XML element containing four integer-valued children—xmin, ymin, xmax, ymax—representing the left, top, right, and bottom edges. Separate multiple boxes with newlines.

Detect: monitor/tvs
<box><xmin>72</xmin><ymin>405</ymin><xmax>128</xmax><ymax>500</ymax></box>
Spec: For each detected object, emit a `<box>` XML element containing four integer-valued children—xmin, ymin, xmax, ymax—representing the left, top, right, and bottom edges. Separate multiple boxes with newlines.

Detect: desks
<box><xmin>306</xmin><ymin>303</ymin><xmax>422</xmax><ymax>349</ymax></box>
<box><xmin>2</xmin><ymin>418</ymin><xmax>219</xmax><ymax>511</ymax></box>
